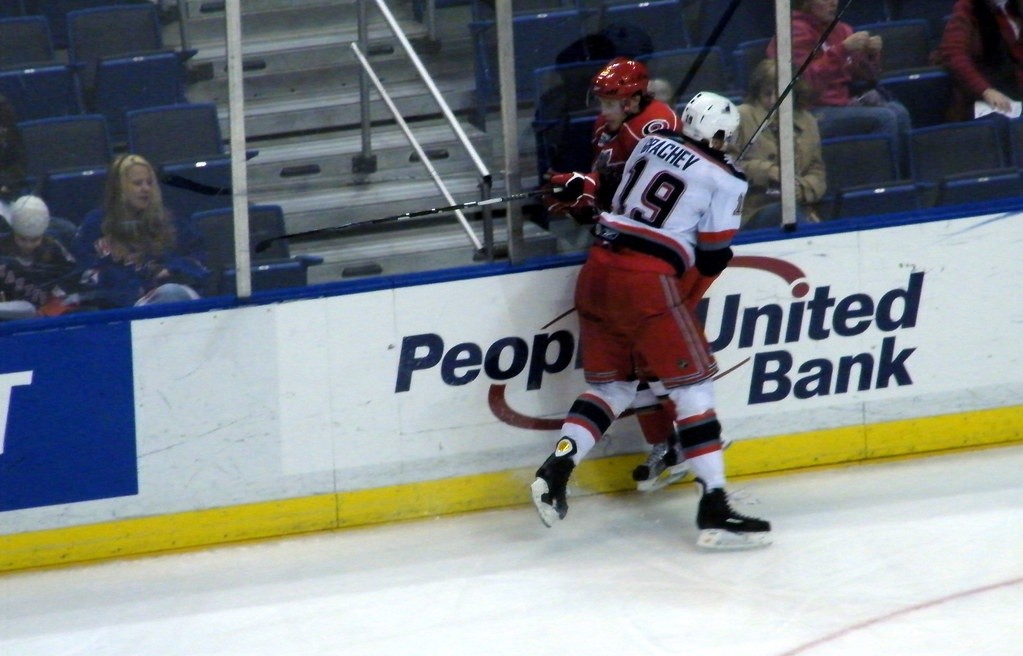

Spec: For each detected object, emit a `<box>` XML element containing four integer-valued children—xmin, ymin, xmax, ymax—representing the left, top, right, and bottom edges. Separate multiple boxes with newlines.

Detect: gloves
<box><xmin>539</xmin><ymin>169</ymin><xmax>600</xmax><ymax>213</ymax></box>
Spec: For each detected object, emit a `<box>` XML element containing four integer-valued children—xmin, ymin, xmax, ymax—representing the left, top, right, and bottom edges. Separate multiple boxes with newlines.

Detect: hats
<box><xmin>11</xmin><ymin>195</ymin><xmax>49</xmax><ymax>238</ymax></box>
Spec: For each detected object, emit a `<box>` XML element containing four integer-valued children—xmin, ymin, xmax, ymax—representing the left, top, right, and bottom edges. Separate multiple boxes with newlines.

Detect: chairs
<box><xmin>462</xmin><ymin>0</ymin><xmax>1023</xmax><ymax>271</ymax></box>
<box><xmin>0</xmin><ymin>0</ymin><xmax>322</xmax><ymax>318</ymax></box>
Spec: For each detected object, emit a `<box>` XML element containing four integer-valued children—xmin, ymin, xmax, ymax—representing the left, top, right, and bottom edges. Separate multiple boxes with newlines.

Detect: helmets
<box><xmin>681</xmin><ymin>91</ymin><xmax>741</xmax><ymax>147</ymax></box>
<box><xmin>591</xmin><ymin>57</ymin><xmax>650</xmax><ymax>99</ymax></box>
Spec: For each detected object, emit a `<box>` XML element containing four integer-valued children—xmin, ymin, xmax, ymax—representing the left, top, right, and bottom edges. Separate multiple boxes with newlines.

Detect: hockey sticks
<box><xmin>667</xmin><ymin>0</ymin><xmax>742</xmax><ymax>110</ymax></box>
<box><xmin>160</xmin><ymin>169</ymin><xmax>500</xmax><ymax>196</ymax></box>
<box><xmin>254</xmin><ymin>185</ymin><xmax>564</xmax><ymax>255</ymax></box>
<box><xmin>734</xmin><ymin>0</ymin><xmax>854</xmax><ymax>166</ymax></box>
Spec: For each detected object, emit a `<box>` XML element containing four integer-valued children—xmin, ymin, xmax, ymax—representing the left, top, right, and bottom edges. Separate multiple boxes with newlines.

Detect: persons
<box><xmin>535</xmin><ymin>59</ymin><xmax>775</xmax><ymax>533</ymax></box>
<box><xmin>716</xmin><ymin>56</ymin><xmax>825</xmax><ymax>231</ymax></box>
<box><xmin>0</xmin><ymin>195</ymin><xmax>76</xmax><ymax>309</ymax></box>
<box><xmin>68</xmin><ymin>151</ymin><xmax>216</xmax><ymax>307</ymax></box>
<box><xmin>766</xmin><ymin>0</ymin><xmax>914</xmax><ymax>186</ymax></box>
<box><xmin>935</xmin><ymin>0</ymin><xmax>1023</xmax><ymax>121</ymax></box>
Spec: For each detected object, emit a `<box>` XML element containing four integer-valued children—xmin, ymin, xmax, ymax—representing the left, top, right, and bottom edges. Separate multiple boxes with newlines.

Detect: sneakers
<box><xmin>691</xmin><ymin>477</ymin><xmax>771</xmax><ymax>548</ymax></box>
<box><xmin>632</xmin><ymin>433</ymin><xmax>689</xmax><ymax>492</ymax></box>
<box><xmin>529</xmin><ymin>436</ymin><xmax>576</xmax><ymax>529</ymax></box>
